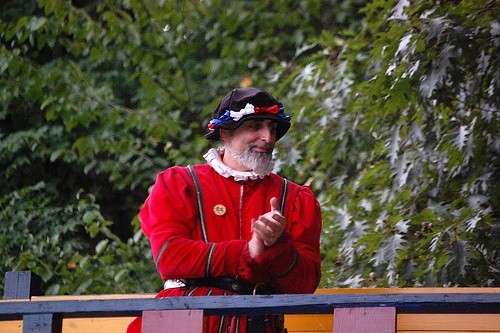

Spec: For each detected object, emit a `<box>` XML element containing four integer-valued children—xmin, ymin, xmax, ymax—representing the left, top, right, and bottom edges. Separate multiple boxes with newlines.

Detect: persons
<box><xmin>126</xmin><ymin>87</ymin><xmax>322</xmax><ymax>333</ymax></box>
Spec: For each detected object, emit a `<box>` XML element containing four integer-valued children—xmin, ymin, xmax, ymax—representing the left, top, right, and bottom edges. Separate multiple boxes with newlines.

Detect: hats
<box><xmin>204</xmin><ymin>88</ymin><xmax>291</xmax><ymax>141</ymax></box>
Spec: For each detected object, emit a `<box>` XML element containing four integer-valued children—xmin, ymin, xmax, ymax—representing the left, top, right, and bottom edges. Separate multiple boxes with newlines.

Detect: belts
<box><xmin>186</xmin><ymin>274</ymin><xmax>277</xmax><ymax>295</ymax></box>
